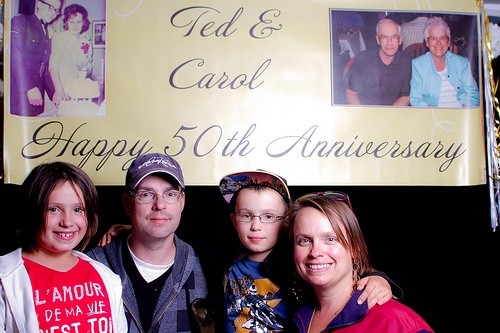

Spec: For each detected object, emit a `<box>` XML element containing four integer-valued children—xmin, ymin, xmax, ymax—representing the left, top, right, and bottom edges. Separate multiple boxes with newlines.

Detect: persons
<box><xmin>9</xmin><ymin>0</ymin><xmax>93</xmax><ymax>117</ymax></box>
<box><xmin>344</xmin><ymin>13</ymin><xmax>479</xmax><ymax>108</ymax></box>
<box><xmin>0</xmin><ymin>153</ymin><xmax>435</xmax><ymax>333</ymax></box>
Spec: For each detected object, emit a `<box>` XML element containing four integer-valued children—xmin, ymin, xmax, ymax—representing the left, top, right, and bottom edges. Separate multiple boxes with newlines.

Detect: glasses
<box><xmin>299</xmin><ymin>192</ymin><xmax>352</xmax><ymax>210</ymax></box>
<box><xmin>38</xmin><ymin>0</ymin><xmax>61</xmax><ymax>15</ymax></box>
<box><xmin>232</xmin><ymin>212</ymin><xmax>285</xmax><ymax>223</ymax></box>
<box><xmin>128</xmin><ymin>190</ymin><xmax>183</xmax><ymax>204</ymax></box>
<box><xmin>427</xmin><ymin>36</ymin><xmax>449</xmax><ymax>41</ymax></box>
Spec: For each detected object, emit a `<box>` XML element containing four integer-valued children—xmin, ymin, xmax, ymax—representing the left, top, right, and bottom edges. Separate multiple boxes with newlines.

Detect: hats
<box><xmin>219</xmin><ymin>169</ymin><xmax>292</xmax><ymax>206</ymax></box>
<box><xmin>126</xmin><ymin>153</ymin><xmax>185</xmax><ymax>191</ymax></box>
